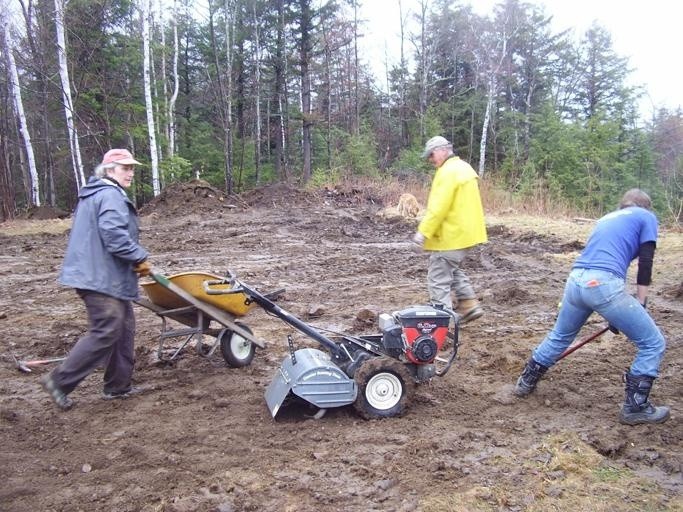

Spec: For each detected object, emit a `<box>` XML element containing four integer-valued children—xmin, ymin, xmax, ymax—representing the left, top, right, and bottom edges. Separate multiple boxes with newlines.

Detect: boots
<box><xmin>619</xmin><ymin>372</ymin><xmax>670</xmax><ymax>425</ymax></box>
<box><xmin>514</xmin><ymin>355</ymin><xmax>548</xmax><ymax>396</ymax></box>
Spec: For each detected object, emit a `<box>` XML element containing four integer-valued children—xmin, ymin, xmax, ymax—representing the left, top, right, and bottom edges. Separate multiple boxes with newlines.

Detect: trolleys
<box><xmin>131</xmin><ymin>261</ymin><xmax>285</xmax><ymax>367</ymax></box>
<box><xmin>203</xmin><ymin>269</ymin><xmax>460</xmax><ymax>420</ymax></box>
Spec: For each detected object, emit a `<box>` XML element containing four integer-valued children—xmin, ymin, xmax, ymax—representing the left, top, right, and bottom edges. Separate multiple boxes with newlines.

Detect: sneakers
<box><xmin>459</xmin><ymin>306</ymin><xmax>483</xmax><ymax>324</ymax></box>
<box><xmin>37</xmin><ymin>372</ymin><xmax>73</xmax><ymax>410</ymax></box>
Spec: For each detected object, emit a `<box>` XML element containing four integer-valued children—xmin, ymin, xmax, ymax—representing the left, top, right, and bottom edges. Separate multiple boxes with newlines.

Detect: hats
<box><xmin>103</xmin><ymin>148</ymin><xmax>144</xmax><ymax>165</ymax></box>
<box><xmin>420</xmin><ymin>136</ymin><xmax>450</xmax><ymax>158</ymax></box>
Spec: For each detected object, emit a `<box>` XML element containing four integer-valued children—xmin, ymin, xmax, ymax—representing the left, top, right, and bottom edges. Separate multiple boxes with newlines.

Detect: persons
<box><xmin>410</xmin><ymin>135</ymin><xmax>488</xmax><ymax>327</ymax></box>
<box><xmin>511</xmin><ymin>187</ymin><xmax>670</xmax><ymax>427</ymax></box>
<box><xmin>39</xmin><ymin>148</ymin><xmax>153</xmax><ymax>410</ymax></box>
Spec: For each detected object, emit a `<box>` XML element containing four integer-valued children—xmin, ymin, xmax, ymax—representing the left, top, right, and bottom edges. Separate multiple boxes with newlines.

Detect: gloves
<box><xmin>132</xmin><ymin>260</ymin><xmax>152</xmax><ymax>277</ymax></box>
<box><xmin>414</xmin><ymin>232</ymin><xmax>424</xmax><ymax>245</ymax></box>
